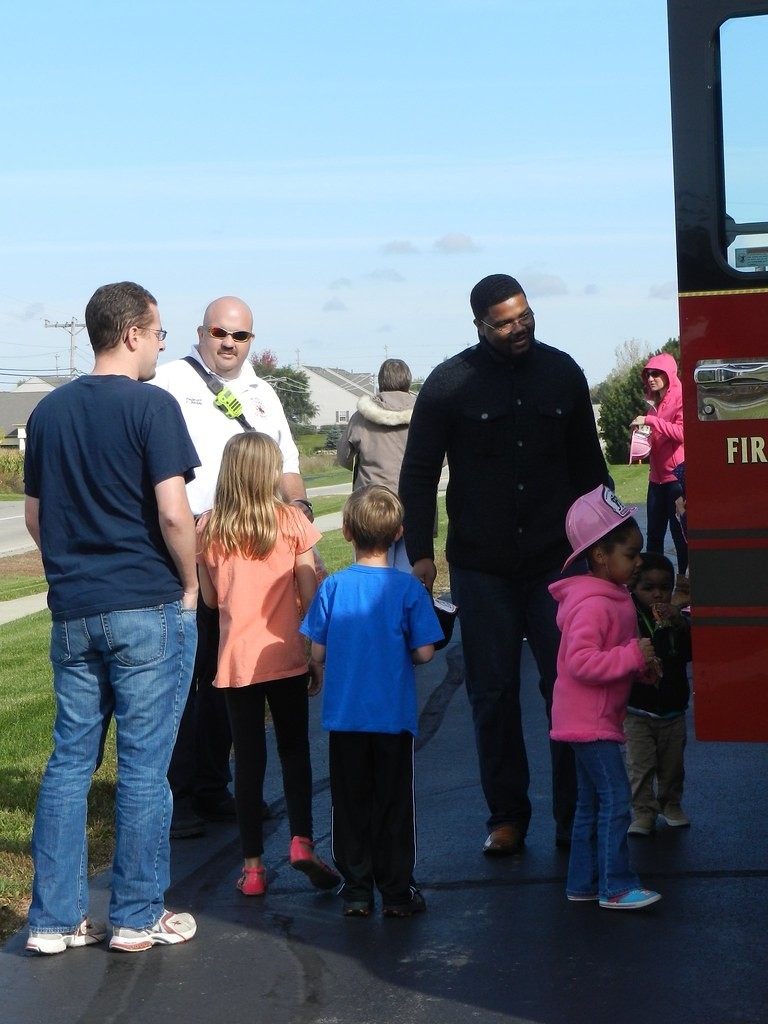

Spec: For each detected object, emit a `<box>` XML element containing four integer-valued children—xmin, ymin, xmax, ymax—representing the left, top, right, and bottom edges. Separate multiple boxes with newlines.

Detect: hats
<box><xmin>628</xmin><ymin>426</ymin><xmax>651</xmax><ymax>468</ymax></box>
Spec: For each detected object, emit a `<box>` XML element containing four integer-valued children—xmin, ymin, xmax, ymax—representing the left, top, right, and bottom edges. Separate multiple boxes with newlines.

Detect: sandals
<box><xmin>288</xmin><ymin>837</ymin><xmax>341</xmax><ymax>890</ymax></box>
<box><xmin>235</xmin><ymin>867</ymin><xmax>269</xmax><ymax>896</ymax></box>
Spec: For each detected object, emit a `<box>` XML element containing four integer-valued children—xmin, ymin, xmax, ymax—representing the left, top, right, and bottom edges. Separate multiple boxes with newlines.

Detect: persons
<box><xmin>621</xmin><ymin>351</ymin><xmax>691</xmax><ymax>576</ymax></box>
<box><xmin>299</xmin><ymin>485</ymin><xmax>444</xmax><ymax>919</ymax></box>
<box><xmin>550</xmin><ymin>486</ymin><xmax>697</xmax><ymax>910</ymax></box>
<box><xmin>23</xmin><ymin>281</ymin><xmax>199</xmax><ymax>955</ymax></box>
<box><xmin>141</xmin><ymin>297</ymin><xmax>340</xmax><ymax>899</ymax></box>
<box><xmin>337</xmin><ymin>359</ymin><xmax>416</xmax><ymax>574</ymax></box>
<box><xmin>399</xmin><ymin>274</ymin><xmax>616</xmax><ymax>857</ymax></box>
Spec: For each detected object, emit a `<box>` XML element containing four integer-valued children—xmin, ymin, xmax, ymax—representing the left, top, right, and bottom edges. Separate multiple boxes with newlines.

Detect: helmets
<box><xmin>558</xmin><ymin>482</ymin><xmax>639</xmax><ymax>575</ymax></box>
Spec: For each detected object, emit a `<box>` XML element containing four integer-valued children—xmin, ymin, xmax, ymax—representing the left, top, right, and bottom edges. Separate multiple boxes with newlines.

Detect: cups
<box><xmin>649</xmin><ymin>624</ymin><xmax>685</xmax><ymax>669</ymax></box>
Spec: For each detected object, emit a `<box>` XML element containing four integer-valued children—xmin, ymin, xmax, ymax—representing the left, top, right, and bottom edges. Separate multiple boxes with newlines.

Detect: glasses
<box><xmin>124</xmin><ymin>326</ymin><xmax>167</xmax><ymax>343</ymax></box>
<box><xmin>202</xmin><ymin>326</ymin><xmax>254</xmax><ymax>343</ymax></box>
<box><xmin>479</xmin><ymin>307</ymin><xmax>535</xmax><ymax>334</ymax></box>
<box><xmin>645</xmin><ymin>369</ymin><xmax>661</xmax><ymax>379</ymax></box>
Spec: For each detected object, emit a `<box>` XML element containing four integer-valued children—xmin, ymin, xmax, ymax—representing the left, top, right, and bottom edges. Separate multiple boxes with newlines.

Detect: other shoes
<box><xmin>171</xmin><ymin>797</ymin><xmax>208</xmax><ymax>836</ymax></box>
<box><xmin>483</xmin><ymin>821</ymin><xmax>526</xmax><ymax>854</ymax></box>
<box><xmin>197</xmin><ymin>792</ymin><xmax>267</xmax><ymax>821</ymax></box>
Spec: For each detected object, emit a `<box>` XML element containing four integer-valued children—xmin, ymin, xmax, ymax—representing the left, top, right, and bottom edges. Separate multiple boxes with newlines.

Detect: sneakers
<box><xmin>382</xmin><ymin>886</ymin><xmax>426</xmax><ymax>917</ymax></box>
<box><xmin>671</xmin><ymin>572</ymin><xmax>691</xmax><ymax>609</ymax></box>
<box><xmin>662</xmin><ymin>804</ymin><xmax>689</xmax><ymax>826</ymax></box>
<box><xmin>628</xmin><ymin>816</ymin><xmax>657</xmax><ymax>834</ymax></box>
<box><xmin>343</xmin><ymin>895</ymin><xmax>375</xmax><ymax>915</ymax></box>
<box><xmin>25</xmin><ymin>915</ymin><xmax>107</xmax><ymax>954</ymax></box>
<box><xmin>599</xmin><ymin>887</ymin><xmax>661</xmax><ymax>908</ymax></box>
<box><xmin>567</xmin><ymin>894</ymin><xmax>601</xmax><ymax>901</ymax></box>
<box><xmin>108</xmin><ymin>908</ymin><xmax>198</xmax><ymax>952</ymax></box>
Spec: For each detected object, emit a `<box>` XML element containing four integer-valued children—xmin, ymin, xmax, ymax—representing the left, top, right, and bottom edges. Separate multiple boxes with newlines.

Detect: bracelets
<box><xmin>184</xmin><ymin>583</ymin><xmax>198</xmax><ymax>594</ymax></box>
<box><xmin>291</xmin><ymin>499</ymin><xmax>313</xmax><ymax>512</ymax></box>
